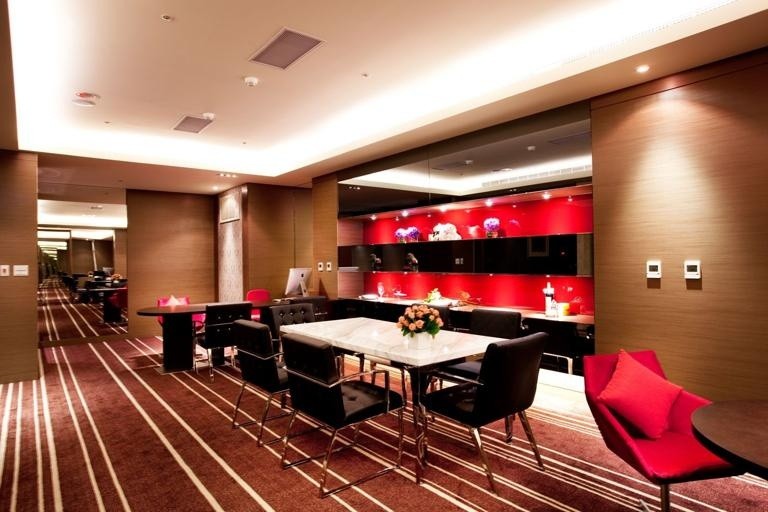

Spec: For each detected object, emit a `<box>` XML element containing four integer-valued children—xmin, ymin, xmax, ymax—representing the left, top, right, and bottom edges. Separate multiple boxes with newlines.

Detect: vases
<box><xmin>487</xmin><ymin>232</ymin><xmax>498</xmax><ymax>238</ymax></box>
<box><xmin>397</xmin><ymin>238</ymin><xmax>405</xmax><ymax>242</ymax></box>
<box><xmin>410</xmin><ymin>237</ymin><xmax>418</xmax><ymax>242</ymax></box>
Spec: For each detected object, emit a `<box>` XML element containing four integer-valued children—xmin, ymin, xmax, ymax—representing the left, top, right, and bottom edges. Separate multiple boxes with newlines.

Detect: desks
<box><xmin>692</xmin><ymin>399</ymin><xmax>768</xmax><ymax>480</ymax></box>
<box><xmin>137</xmin><ymin>301</ymin><xmax>289</xmax><ymax>369</ymax></box>
<box><xmin>280</xmin><ymin>316</ymin><xmax>511</xmax><ymax>484</ymax></box>
<box><xmin>89</xmin><ymin>288</ymin><xmax>120</xmax><ymax>322</ymax></box>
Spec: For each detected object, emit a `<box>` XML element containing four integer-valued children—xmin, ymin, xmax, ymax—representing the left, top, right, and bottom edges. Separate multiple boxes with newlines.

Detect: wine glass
<box><xmin>378</xmin><ymin>282</ymin><xmax>384</xmax><ymax>302</ymax></box>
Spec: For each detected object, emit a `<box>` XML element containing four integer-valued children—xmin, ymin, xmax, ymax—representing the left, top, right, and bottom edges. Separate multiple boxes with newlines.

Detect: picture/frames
<box><xmin>527</xmin><ymin>237</ymin><xmax>549</xmax><ymax>257</ymax></box>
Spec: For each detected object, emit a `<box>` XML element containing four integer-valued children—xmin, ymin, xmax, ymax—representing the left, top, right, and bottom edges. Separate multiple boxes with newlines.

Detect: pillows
<box><xmin>597</xmin><ymin>349</ymin><xmax>683</xmax><ymax>439</ymax></box>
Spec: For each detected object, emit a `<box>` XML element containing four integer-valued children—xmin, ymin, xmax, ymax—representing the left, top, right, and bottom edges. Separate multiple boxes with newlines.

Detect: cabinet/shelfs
<box><xmin>338</xmin><ymin>235</ymin><xmax>593</xmax><ymax>375</ymax></box>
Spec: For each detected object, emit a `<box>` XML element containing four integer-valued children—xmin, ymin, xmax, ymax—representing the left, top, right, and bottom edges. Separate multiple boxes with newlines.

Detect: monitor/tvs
<box><xmin>103</xmin><ymin>267</ymin><xmax>113</xmax><ymax>276</ymax></box>
<box><xmin>284</xmin><ymin>267</ymin><xmax>313</xmax><ymax>297</ymax></box>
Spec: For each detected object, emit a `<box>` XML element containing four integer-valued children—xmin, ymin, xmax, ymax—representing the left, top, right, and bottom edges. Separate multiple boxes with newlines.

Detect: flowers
<box><xmin>395</xmin><ymin>228</ymin><xmax>406</xmax><ymax>238</ymax></box>
<box><xmin>484</xmin><ymin>218</ymin><xmax>500</xmax><ymax>231</ymax></box>
<box><xmin>408</xmin><ymin>226</ymin><xmax>420</xmax><ymax>238</ymax></box>
<box><xmin>368</xmin><ymin>253</ymin><xmax>381</xmax><ymax>270</ymax></box>
<box><xmin>399</xmin><ymin>306</ymin><xmax>444</xmax><ymax>338</ymax></box>
<box><xmin>404</xmin><ymin>252</ymin><xmax>419</xmax><ymax>271</ymax></box>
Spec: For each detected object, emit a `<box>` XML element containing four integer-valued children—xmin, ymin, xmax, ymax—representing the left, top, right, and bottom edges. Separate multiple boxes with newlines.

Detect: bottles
<box><xmin>546</xmin><ymin>282</ymin><xmax>553</xmax><ymax>316</ymax></box>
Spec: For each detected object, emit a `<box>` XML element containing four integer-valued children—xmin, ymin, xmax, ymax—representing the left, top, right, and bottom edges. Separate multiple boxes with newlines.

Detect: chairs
<box><xmin>426</xmin><ymin>332</ymin><xmax>548</xmax><ymax>497</ymax></box>
<box><xmin>193</xmin><ymin>303</ymin><xmax>254</xmax><ymax>369</ymax></box>
<box><xmin>279</xmin><ymin>333</ymin><xmax>402</xmax><ymax>498</ymax></box>
<box><xmin>411</xmin><ymin>305</ymin><xmax>448</xmax><ymax>390</ymax></box>
<box><xmin>270</xmin><ymin>302</ymin><xmax>316</xmax><ymax>353</ymax></box>
<box><xmin>232</xmin><ymin>319</ymin><xmax>323</xmax><ymax>449</ymax></box>
<box><xmin>582</xmin><ymin>350</ymin><xmax>746</xmax><ymax>512</ymax></box>
<box><xmin>444</xmin><ymin>310</ymin><xmax>521</xmax><ymax>420</ymax></box>
<box><xmin>69</xmin><ymin>274</ymin><xmax>128</xmax><ymax>308</ymax></box>
